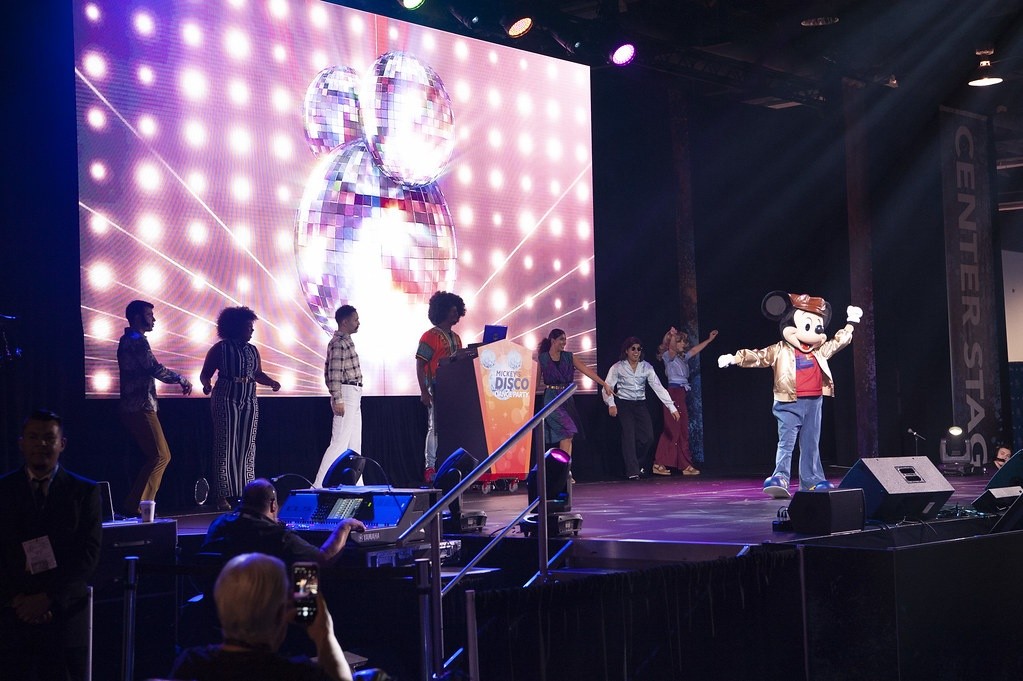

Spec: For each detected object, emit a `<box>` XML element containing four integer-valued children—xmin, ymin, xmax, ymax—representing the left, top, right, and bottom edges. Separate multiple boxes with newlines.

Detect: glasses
<box><xmin>630</xmin><ymin>347</ymin><xmax>643</xmax><ymax>352</ymax></box>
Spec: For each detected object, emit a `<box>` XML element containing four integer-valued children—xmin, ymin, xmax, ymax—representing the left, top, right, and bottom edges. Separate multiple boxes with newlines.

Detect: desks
<box><xmin>382</xmin><ymin>568</ymin><xmax>499</xmax><ymax>681</ymax></box>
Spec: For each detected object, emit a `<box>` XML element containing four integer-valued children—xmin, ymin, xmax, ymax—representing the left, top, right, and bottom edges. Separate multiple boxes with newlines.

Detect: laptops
<box><xmin>483</xmin><ymin>325</ymin><xmax>507</xmax><ymax>343</ymax></box>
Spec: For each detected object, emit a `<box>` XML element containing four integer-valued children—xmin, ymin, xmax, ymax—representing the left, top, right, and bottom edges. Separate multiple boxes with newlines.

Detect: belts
<box><xmin>342</xmin><ymin>381</ymin><xmax>362</xmax><ymax>386</ymax></box>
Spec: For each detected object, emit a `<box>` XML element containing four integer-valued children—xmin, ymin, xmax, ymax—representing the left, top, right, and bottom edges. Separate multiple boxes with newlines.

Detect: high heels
<box><xmin>683</xmin><ymin>465</ymin><xmax>701</xmax><ymax>475</ymax></box>
<box><xmin>653</xmin><ymin>464</ymin><xmax>672</xmax><ymax>475</ymax></box>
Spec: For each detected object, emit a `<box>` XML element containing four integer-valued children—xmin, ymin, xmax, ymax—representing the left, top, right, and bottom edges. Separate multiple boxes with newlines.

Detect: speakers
<box><xmin>787</xmin><ymin>456</ymin><xmax>955</xmax><ymax>536</ymax></box>
<box><xmin>971</xmin><ymin>448</ymin><xmax>1023</xmax><ymax>514</ymax></box>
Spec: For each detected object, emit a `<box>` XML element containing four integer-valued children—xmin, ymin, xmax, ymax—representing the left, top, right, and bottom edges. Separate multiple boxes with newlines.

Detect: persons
<box><xmin>310</xmin><ymin>305</ymin><xmax>365</xmax><ymax>490</ymax></box>
<box><xmin>652</xmin><ymin>327</ymin><xmax>718</xmax><ymax>476</ymax></box>
<box><xmin>718</xmin><ymin>291</ymin><xmax>863</xmax><ymax>497</ymax></box>
<box><xmin>117</xmin><ymin>300</ymin><xmax>192</xmax><ymax>518</ymax></box>
<box><xmin>197</xmin><ymin>479</ymin><xmax>367</xmax><ymax>656</ymax></box>
<box><xmin>200</xmin><ymin>306</ymin><xmax>281</xmax><ymax>511</ymax></box>
<box><xmin>601</xmin><ymin>337</ymin><xmax>680</xmax><ymax>481</ymax></box>
<box><xmin>536</xmin><ymin>328</ymin><xmax>617</xmax><ymax>484</ymax></box>
<box><xmin>416</xmin><ymin>291</ymin><xmax>467</xmax><ymax>482</ymax></box>
<box><xmin>169</xmin><ymin>553</ymin><xmax>352</xmax><ymax>680</ymax></box>
<box><xmin>0</xmin><ymin>411</ymin><xmax>103</xmax><ymax>681</ymax></box>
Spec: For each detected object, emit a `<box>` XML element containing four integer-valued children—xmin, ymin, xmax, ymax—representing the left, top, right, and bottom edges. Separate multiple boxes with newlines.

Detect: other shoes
<box><xmin>215</xmin><ymin>499</ymin><xmax>232</xmax><ymax>511</ymax></box>
<box><xmin>624</xmin><ymin>467</ymin><xmax>651</xmax><ymax>480</ymax></box>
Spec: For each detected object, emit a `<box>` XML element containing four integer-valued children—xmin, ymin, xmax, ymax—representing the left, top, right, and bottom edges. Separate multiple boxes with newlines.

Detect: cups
<box><xmin>139</xmin><ymin>500</ymin><xmax>156</xmax><ymax>522</ymax></box>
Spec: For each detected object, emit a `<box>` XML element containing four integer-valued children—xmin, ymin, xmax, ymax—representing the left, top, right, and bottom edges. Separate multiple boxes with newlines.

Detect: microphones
<box><xmin>908</xmin><ymin>428</ymin><xmax>917</xmax><ymax>435</ymax></box>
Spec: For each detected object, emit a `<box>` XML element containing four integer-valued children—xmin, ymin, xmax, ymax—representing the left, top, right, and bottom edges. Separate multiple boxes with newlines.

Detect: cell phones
<box><xmin>291</xmin><ymin>561</ymin><xmax>320</xmax><ymax>624</ymax></box>
